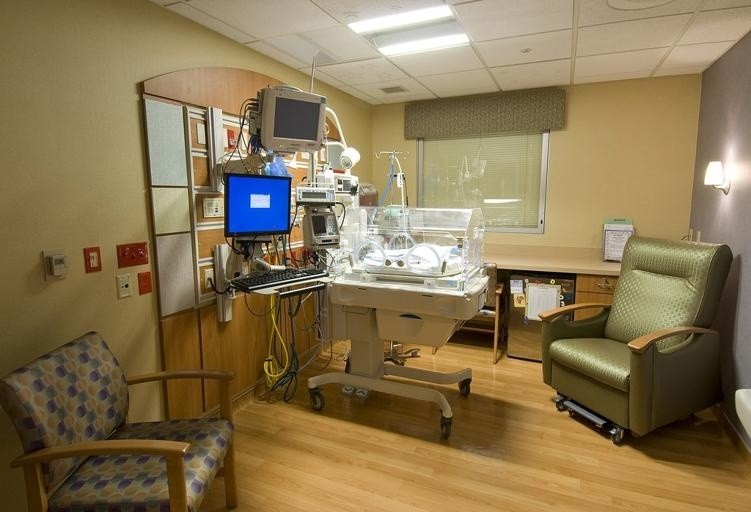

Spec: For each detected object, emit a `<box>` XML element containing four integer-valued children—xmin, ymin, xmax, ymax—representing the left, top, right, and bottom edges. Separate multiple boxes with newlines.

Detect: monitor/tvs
<box><xmin>260</xmin><ymin>88</ymin><xmax>327</xmax><ymax>151</ymax></box>
<box><xmin>224</xmin><ymin>173</ymin><xmax>292</xmax><ymax>236</ymax></box>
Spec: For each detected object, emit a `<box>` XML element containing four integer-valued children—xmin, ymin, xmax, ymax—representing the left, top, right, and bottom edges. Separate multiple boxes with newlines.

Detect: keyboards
<box><xmin>231</xmin><ymin>268</ymin><xmax>328</xmax><ymax>290</ymax></box>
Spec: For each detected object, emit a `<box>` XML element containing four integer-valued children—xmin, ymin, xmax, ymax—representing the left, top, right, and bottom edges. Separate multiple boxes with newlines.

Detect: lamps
<box><xmin>703</xmin><ymin>160</ymin><xmax>733</xmax><ymax>196</ymax></box>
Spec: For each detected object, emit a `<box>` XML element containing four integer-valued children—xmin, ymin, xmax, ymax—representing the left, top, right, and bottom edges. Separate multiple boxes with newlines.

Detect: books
<box><xmin>602</xmin><ymin>217</ymin><xmax>636</xmax><ymax>262</ymax></box>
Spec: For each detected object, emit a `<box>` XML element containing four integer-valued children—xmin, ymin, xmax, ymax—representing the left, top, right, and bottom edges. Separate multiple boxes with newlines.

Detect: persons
<box><xmin>335</xmin><ymin>183</ymin><xmax>399</xmax><ymax>259</ymax></box>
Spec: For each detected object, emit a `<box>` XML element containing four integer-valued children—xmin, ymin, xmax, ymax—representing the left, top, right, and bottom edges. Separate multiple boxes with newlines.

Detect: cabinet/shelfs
<box><xmin>574</xmin><ymin>276</ymin><xmax>621</xmax><ymax>321</ymax></box>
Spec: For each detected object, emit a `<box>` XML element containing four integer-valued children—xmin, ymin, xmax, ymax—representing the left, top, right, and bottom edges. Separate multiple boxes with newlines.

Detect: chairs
<box><xmin>481</xmin><ymin>263</ymin><xmax>506</xmax><ymax>363</ymax></box>
<box><xmin>1</xmin><ymin>330</ymin><xmax>241</xmax><ymax>512</ymax></box>
<box><xmin>538</xmin><ymin>233</ymin><xmax>733</xmax><ymax>444</ymax></box>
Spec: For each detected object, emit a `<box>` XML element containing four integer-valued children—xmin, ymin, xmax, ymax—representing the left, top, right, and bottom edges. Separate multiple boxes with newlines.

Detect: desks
<box><xmin>484</xmin><ymin>244</ymin><xmax>624</xmax><ymax>277</ymax></box>
